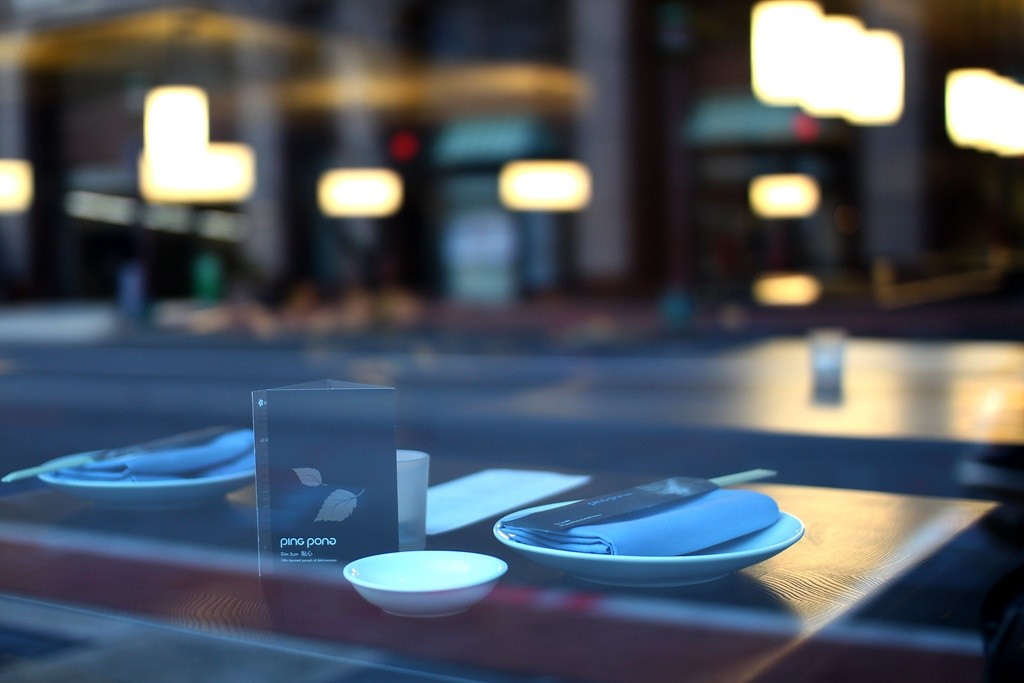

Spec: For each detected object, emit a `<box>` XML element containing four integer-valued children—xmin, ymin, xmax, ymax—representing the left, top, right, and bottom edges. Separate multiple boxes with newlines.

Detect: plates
<box><xmin>492</xmin><ymin>498</ymin><xmax>806</xmax><ymax>588</ymax></box>
<box><xmin>37</xmin><ymin>451</ymin><xmax>255</xmax><ymax>514</ymax></box>
<box><xmin>425</xmin><ymin>469</ymin><xmax>592</xmax><ymax>535</ymax></box>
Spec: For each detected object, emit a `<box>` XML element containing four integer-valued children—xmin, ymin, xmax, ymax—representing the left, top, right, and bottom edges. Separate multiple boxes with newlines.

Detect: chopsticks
<box><xmin>709</xmin><ymin>469</ymin><xmax>778</xmax><ymax>486</ymax></box>
<box><xmin>1</xmin><ymin>451</ymin><xmax>91</xmax><ymax>484</ymax></box>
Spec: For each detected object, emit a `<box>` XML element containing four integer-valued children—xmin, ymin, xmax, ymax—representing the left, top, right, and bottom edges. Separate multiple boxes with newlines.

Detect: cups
<box><xmin>810</xmin><ymin>333</ymin><xmax>843</xmax><ymax>388</ymax></box>
<box><xmin>396</xmin><ymin>449</ymin><xmax>429</xmax><ymax>551</ymax></box>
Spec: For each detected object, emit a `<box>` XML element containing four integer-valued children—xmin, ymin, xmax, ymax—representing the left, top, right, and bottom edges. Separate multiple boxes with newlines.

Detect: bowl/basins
<box><xmin>344</xmin><ymin>551</ymin><xmax>509</xmax><ymax>618</ymax></box>
